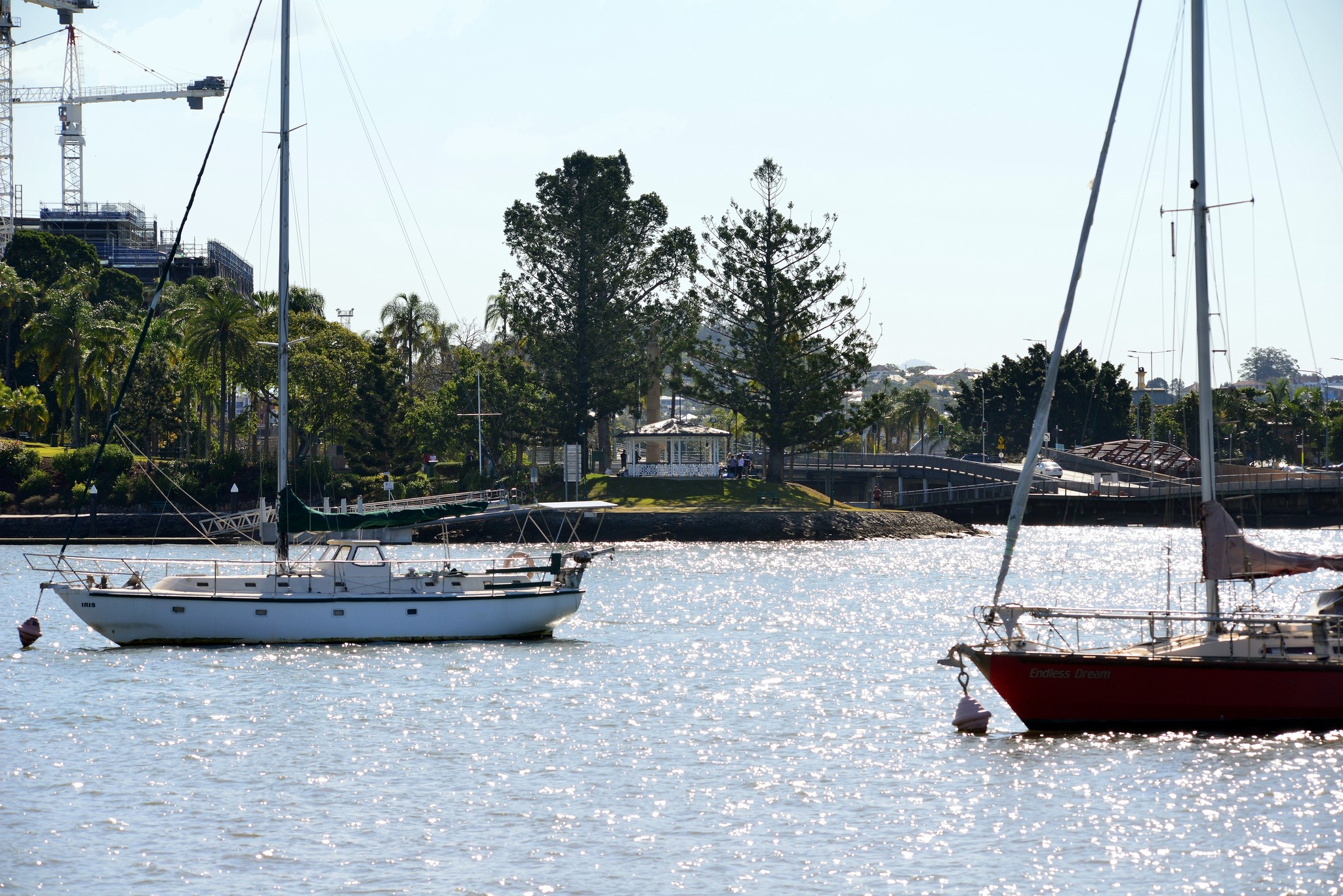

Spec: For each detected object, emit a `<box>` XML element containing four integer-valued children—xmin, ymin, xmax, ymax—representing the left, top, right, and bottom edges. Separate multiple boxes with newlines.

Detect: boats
<box><xmin>1306</xmin><ymin>463</ymin><xmax>1343</xmax><ymax>472</ymax></box>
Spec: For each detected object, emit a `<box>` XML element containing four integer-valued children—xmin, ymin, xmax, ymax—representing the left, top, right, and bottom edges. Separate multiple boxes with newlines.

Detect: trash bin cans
<box><xmin>722</xmin><ymin>473</ymin><xmax>728</xmax><ymax>478</ymax></box>
<box><xmin>727</xmin><ymin>474</ymin><xmax>734</xmax><ymax>478</ymax></box>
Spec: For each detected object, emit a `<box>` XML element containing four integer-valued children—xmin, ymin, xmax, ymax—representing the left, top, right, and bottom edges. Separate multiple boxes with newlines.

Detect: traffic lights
<box><xmin>871</xmin><ymin>422</ymin><xmax>877</xmax><ymax>434</ymax></box>
<box><xmin>859</xmin><ymin>431</ymin><xmax>863</xmax><ymax>436</ymax></box>
<box><xmin>897</xmin><ymin>436</ymin><xmax>900</xmax><ymax>444</ymax></box>
<box><xmin>938</xmin><ymin>424</ymin><xmax>944</xmax><ymax>436</ymax></box>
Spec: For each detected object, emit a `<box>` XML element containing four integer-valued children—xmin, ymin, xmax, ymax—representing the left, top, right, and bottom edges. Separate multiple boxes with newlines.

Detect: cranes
<box><xmin>0</xmin><ymin>0</ymin><xmax>230</xmax><ymax>263</ymax></box>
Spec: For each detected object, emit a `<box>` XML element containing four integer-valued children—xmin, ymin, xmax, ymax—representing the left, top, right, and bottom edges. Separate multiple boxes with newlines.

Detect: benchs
<box><xmin>61</xmin><ymin>442</ymin><xmax>82</xmax><ymax>454</ymax></box>
<box><xmin>749</xmin><ymin>470</ymin><xmax>761</xmax><ymax>479</ymax></box>
<box><xmin>756</xmin><ymin>490</ymin><xmax>782</xmax><ymax>504</ymax></box>
<box><xmin>149</xmin><ymin>501</ymin><xmax>176</xmax><ymax>512</ymax></box>
<box><xmin>152</xmin><ymin>451</ymin><xmax>189</xmax><ymax>455</ymax></box>
<box><xmin>19</xmin><ymin>431</ymin><xmax>34</xmax><ymax>442</ymax></box>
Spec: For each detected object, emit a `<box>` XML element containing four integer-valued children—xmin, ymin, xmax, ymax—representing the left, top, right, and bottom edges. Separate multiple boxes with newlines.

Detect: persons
<box><xmin>885</xmin><ymin>451</ymin><xmax>888</xmax><ymax>454</ymax></box>
<box><xmin>726</xmin><ymin>452</ymin><xmax>751</xmax><ymax>479</ymax></box>
<box><xmin>604</xmin><ymin>468</ymin><xmax>613</xmax><ymax>475</ymax></box>
<box><xmin>423</xmin><ymin>450</ymin><xmax>494</xmax><ymax>478</ymax></box>
<box><xmin>871</xmin><ymin>486</ymin><xmax>882</xmax><ymax>509</ymax></box>
<box><xmin>621</xmin><ymin>450</ymin><xmax>627</xmax><ymax>470</ymax></box>
<box><xmin>631</xmin><ymin>449</ymin><xmax>641</xmax><ymax>465</ymax></box>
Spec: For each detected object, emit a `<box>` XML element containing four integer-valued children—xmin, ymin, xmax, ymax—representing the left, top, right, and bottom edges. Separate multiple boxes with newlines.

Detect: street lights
<box><xmin>981</xmin><ymin>387</ymin><xmax>985</xmax><ymax>464</ymax></box>
<box><xmin>1024</xmin><ymin>338</ymin><xmax>1049</xmax><ymax>460</ymax></box>
<box><xmin>1127</xmin><ymin>349</ymin><xmax>1175</xmax><ymax>479</ymax></box>
<box><xmin>732</xmin><ymin>410</ymin><xmax>738</xmax><ymax>460</ymax></box>
<box><xmin>1290</xmin><ymin>369</ymin><xmax>1329</xmax><ymax>472</ymax></box>
<box><xmin>590</xmin><ymin>441</ymin><xmax>599</xmax><ymax>470</ymax></box>
<box><xmin>830</xmin><ymin>422</ymin><xmax>846</xmax><ymax>508</ymax></box>
<box><xmin>1127</xmin><ymin>355</ymin><xmax>1139</xmax><ymax>445</ymax></box>
<box><xmin>710</xmin><ymin>418</ymin><xmax>717</xmax><ymax>462</ymax></box>
<box><xmin>700</xmin><ymin>437</ymin><xmax>709</xmax><ymax>463</ymax></box>
<box><xmin>885</xmin><ymin>412</ymin><xmax>895</xmax><ymax>454</ymax></box>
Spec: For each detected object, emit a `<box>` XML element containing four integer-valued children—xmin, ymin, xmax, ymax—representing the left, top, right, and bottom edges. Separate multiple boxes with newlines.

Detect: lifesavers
<box><xmin>503</xmin><ymin>552</ymin><xmax>535</xmax><ymax>579</ymax></box>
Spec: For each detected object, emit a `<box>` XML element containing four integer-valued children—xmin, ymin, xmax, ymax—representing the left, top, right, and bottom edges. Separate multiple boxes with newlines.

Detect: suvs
<box><xmin>961</xmin><ymin>453</ymin><xmax>1006</xmax><ymax>464</ymax></box>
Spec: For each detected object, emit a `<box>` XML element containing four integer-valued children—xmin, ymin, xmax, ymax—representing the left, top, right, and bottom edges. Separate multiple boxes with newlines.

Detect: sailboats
<box><xmin>927</xmin><ymin>0</ymin><xmax>1342</xmax><ymax>743</ymax></box>
<box><xmin>24</xmin><ymin>0</ymin><xmax>620</xmax><ymax>639</ymax></box>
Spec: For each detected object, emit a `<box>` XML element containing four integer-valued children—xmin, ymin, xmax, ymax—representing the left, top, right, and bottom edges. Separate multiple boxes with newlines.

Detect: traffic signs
<box><xmin>892</xmin><ymin>437</ymin><xmax>896</xmax><ymax>444</ymax></box>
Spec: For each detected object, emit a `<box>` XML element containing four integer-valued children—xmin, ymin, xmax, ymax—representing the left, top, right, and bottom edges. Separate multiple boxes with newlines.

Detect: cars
<box><xmin>1281</xmin><ymin>466</ymin><xmax>1308</xmax><ymax>473</ymax></box>
<box><xmin>1035</xmin><ymin>460</ymin><xmax>1063</xmax><ymax>478</ymax></box>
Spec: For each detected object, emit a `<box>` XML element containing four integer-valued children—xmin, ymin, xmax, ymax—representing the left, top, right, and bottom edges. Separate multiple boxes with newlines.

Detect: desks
<box><xmin>155</xmin><ymin>447</ymin><xmax>186</xmax><ymax>459</ymax></box>
